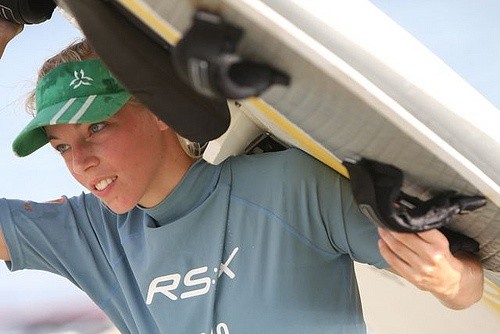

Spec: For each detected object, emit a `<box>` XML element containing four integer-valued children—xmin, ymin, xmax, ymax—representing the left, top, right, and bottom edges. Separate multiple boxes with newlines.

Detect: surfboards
<box><xmin>56</xmin><ymin>0</ymin><xmax>499</xmax><ymax>274</ymax></box>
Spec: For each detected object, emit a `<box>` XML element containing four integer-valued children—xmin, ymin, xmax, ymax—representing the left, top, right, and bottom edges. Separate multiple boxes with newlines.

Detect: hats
<box><xmin>10</xmin><ymin>58</ymin><xmax>131</xmax><ymax>157</ymax></box>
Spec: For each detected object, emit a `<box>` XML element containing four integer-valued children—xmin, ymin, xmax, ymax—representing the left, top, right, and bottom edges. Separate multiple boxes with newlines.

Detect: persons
<box><xmin>0</xmin><ymin>24</ymin><xmax>485</xmax><ymax>334</ymax></box>
